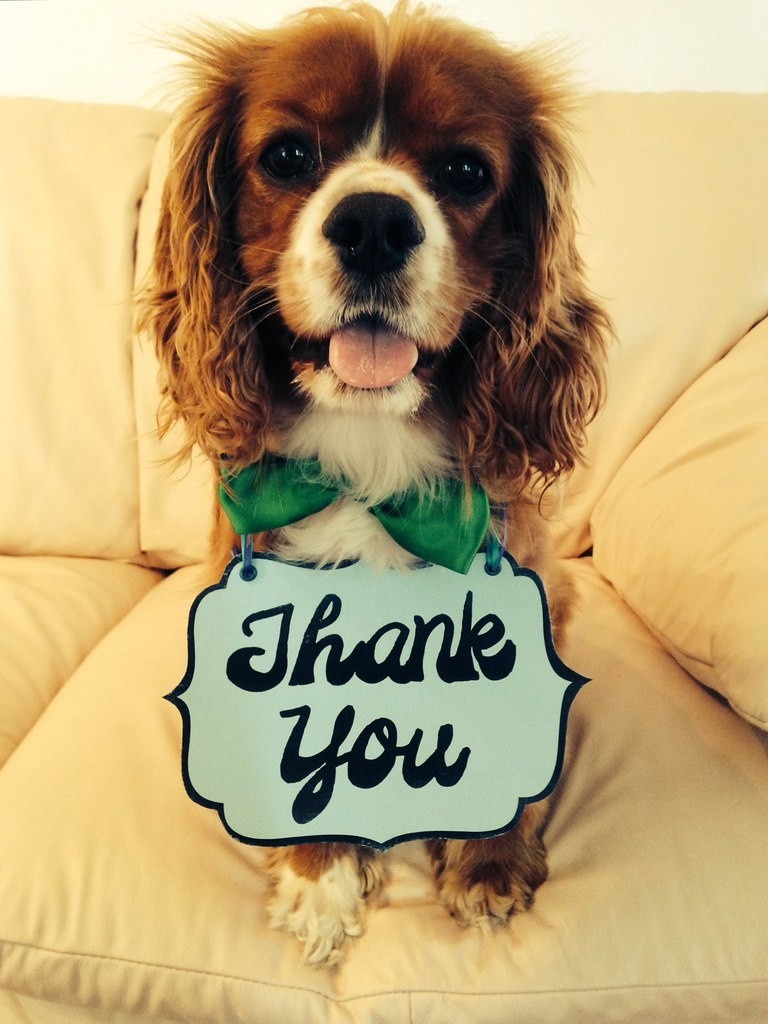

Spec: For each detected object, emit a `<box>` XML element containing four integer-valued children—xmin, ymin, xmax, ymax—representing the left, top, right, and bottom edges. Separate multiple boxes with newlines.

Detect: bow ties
<box><xmin>218</xmin><ymin>453</ymin><xmax>489</xmax><ymax>575</ymax></box>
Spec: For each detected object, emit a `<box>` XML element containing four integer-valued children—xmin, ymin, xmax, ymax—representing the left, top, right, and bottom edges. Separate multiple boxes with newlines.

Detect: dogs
<box><xmin>135</xmin><ymin>0</ymin><xmax>619</xmax><ymax>968</ymax></box>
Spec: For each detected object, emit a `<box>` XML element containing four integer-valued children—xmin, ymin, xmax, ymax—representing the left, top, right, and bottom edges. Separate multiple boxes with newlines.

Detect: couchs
<box><xmin>0</xmin><ymin>93</ymin><xmax>768</xmax><ymax>1024</ymax></box>
